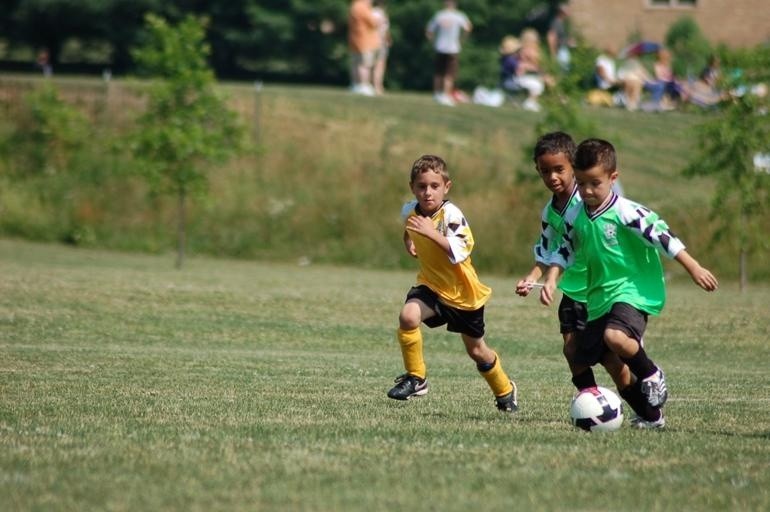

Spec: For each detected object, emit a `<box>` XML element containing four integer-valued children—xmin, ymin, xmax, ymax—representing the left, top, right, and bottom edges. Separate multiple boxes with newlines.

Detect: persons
<box><xmin>382</xmin><ymin>153</ymin><xmax>519</xmax><ymax>415</ymax></box>
<box><xmin>496</xmin><ymin>2</ymin><xmax>766</xmax><ymax>114</ymax></box>
<box><xmin>348</xmin><ymin>1</ymin><xmax>387</xmax><ymax>97</ymax></box>
<box><xmin>542</xmin><ymin>138</ymin><xmax>720</xmax><ymax>429</ymax></box>
<box><xmin>513</xmin><ymin>131</ymin><xmax>613</xmax><ymax>390</ymax></box>
<box><xmin>372</xmin><ymin>1</ymin><xmax>394</xmax><ymax>96</ymax></box>
<box><xmin>422</xmin><ymin>1</ymin><xmax>475</xmax><ymax>108</ymax></box>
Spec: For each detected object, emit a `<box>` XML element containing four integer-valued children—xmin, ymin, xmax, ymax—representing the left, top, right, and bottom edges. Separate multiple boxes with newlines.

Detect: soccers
<box><xmin>570</xmin><ymin>387</ymin><xmax>624</xmax><ymax>432</ymax></box>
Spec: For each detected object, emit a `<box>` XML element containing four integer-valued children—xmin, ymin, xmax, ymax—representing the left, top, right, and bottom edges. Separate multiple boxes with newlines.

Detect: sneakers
<box><xmin>641</xmin><ymin>365</ymin><xmax>668</xmax><ymax>409</ymax></box>
<box><xmin>388</xmin><ymin>373</ymin><xmax>428</xmax><ymax>398</ymax></box>
<box><xmin>629</xmin><ymin>409</ymin><xmax>666</xmax><ymax>430</ymax></box>
<box><xmin>496</xmin><ymin>381</ymin><xmax>518</xmax><ymax>413</ymax></box>
